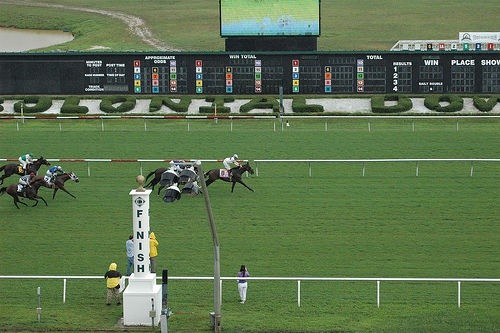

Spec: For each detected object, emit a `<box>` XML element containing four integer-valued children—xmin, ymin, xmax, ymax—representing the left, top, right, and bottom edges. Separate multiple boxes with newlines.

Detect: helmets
<box><xmin>57</xmin><ymin>166</ymin><xmax>61</xmax><ymax>170</ymax></box>
<box><xmin>29</xmin><ymin>153</ymin><xmax>33</xmax><ymax>157</ymax></box>
<box><xmin>196</xmin><ymin>160</ymin><xmax>201</xmax><ymax>166</ymax></box>
<box><xmin>233</xmin><ymin>154</ymin><xmax>238</xmax><ymax>159</ymax></box>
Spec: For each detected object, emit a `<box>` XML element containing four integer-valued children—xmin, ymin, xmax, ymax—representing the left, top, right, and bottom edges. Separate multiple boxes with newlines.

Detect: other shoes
<box><xmin>240</xmin><ymin>300</ymin><xmax>245</xmax><ymax>305</ymax></box>
<box><xmin>107</xmin><ymin>304</ymin><xmax>110</xmax><ymax>305</ymax></box>
<box><xmin>117</xmin><ymin>302</ymin><xmax>120</xmax><ymax>305</ymax></box>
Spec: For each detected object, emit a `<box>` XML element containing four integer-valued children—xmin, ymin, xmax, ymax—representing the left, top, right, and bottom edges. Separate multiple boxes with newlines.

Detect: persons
<box><xmin>19</xmin><ymin>153</ymin><xmax>33</xmax><ymax>169</ymax></box>
<box><xmin>149</xmin><ymin>232</ymin><xmax>159</xmax><ymax>273</ymax></box>
<box><xmin>19</xmin><ymin>173</ymin><xmax>35</xmax><ymax>187</ymax></box>
<box><xmin>125</xmin><ymin>235</ymin><xmax>134</xmax><ymax>276</ymax></box>
<box><xmin>170</xmin><ymin>160</ymin><xmax>201</xmax><ymax>173</ymax></box>
<box><xmin>224</xmin><ymin>153</ymin><xmax>241</xmax><ymax>177</ymax></box>
<box><xmin>104</xmin><ymin>263</ymin><xmax>121</xmax><ymax>306</ymax></box>
<box><xmin>237</xmin><ymin>265</ymin><xmax>250</xmax><ymax>304</ymax></box>
<box><xmin>47</xmin><ymin>166</ymin><xmax>65</xmax><ymax>177</ymax></box>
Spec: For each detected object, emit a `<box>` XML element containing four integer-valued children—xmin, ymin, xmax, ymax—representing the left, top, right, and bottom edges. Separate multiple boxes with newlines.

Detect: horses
<box><xmin>0</xmin><ymin>176</ymin><xmax>52</xmax><ymax>209</ymax></box>
<box><xmin>204</xmin><ymin>161</ymin><xmax>255</xmax><ymax>193</ymax></box>
<box><xmin>0</xmin><ymin>156</ymin><xmax>51</xmax><ymax>185</ymax></box>
<box><xmin>143</xmin><ymin>166</ymin><xmax>197</xmax><ymax>190</ymax></box>
<box><xmin>42</xmin><ymin>170</ymin><xmax>79</xmax><ymax>198</ymax></box>
